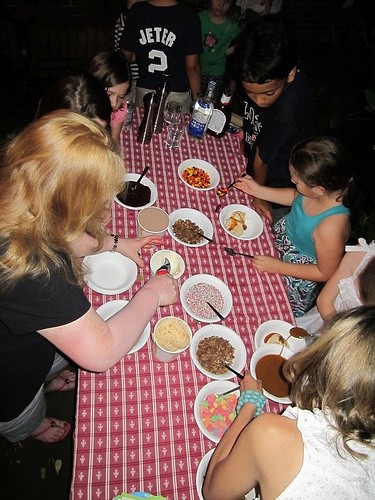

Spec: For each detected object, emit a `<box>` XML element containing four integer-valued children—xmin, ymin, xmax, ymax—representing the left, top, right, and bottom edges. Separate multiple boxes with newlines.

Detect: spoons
<box><xmin>154</xmin><ymin>258</ymin><xmax>171</xmax><ymax>307</ymax></box>
<box><xmin>224</xmin><ymin>248</ymin><xmax>254</xmax><ymax>258</ymax></box>
<box><xmin>289</xmin><ymin>327</ymin><xmax>323</xmax><ymax>340</ymax></box>
<box><xmin>216</xmin><ymin>173</ymin><xmax>246</xmax><ymax>197</ymax></box>
<box><xmin>206</xmin><ymin>385</ymin><xmax>241</xmax><ymax>400</ymax></box>
<box><xmin>130</xmin><ymin>165</ymin><xmax>149</xmax><ymax>194</ymax></box>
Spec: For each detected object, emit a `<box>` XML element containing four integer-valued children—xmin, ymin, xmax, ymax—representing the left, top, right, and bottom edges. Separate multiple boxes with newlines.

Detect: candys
<box><xmin>201</xmin><ymin>394</ymin><xmax>238</xmax><ymax>437</ymax></box>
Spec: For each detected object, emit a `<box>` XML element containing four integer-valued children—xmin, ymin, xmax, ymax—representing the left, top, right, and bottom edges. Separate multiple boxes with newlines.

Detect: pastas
<box><xmin>156</xmin><ymin>321</ymin><xmax>189</xmax><ymax>351</ymax></box>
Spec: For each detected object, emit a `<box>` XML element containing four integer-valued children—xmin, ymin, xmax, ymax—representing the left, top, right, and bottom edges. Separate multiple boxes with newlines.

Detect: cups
<box><xmin>162</xmin><ymin>100</ymin><xmax>182</xmax><ymax>124</ymax></box>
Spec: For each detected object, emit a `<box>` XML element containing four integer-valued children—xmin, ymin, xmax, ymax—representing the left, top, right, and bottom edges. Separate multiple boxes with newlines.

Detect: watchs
<box><xmin>109</xmin><ymin>233</ymin><xmax>120</xmax><ymax>253</ymax></box>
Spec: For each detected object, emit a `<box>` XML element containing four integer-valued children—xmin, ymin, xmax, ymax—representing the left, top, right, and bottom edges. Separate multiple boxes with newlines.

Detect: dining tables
<box><xmin>67</xmin><ymin>105</ymin><xmax>305</xmax><ymax>500</ymax></box>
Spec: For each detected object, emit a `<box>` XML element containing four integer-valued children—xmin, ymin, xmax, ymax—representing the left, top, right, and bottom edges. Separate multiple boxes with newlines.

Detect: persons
<box><xmin>292</xmin><ymin>237</ymin><xmax>375</xmax><ymax>350</ymax></box>
<box><xmin>114</xmin><ymin>0</ymin><xmax>201</xmax><ymax>109</ymax></box>
<box><xmin>0</xmin><ymin>109</ymin><xmax>180</xmax><ymax>442</ymax></box>
<box><xmin>13</xmin><ymin>73</ymin><xmax>111</xmax><ymax>144</ymax></box>
<box><xmin>202</xmin><ymin>306</ymin><xmax>373</xmax><ymax>500</ymax></box>
<box><xmin>80</xmin><ymin>51</ymin><xmax>132</xmax><ymax>147</ymax></box>
<box><xmin>231</xmin><ymin>138</ymin><xmax>355</xmax><ymax>318</ymax></box>
<box><xmin>224</xmin><ymin>32</ymin><xmax>320</xmax><ymax>226</ymax></box>
<box><xmin>196</xmin><ymin>0</ymin><xmax>242</xmax><ymax>102</ymax></box>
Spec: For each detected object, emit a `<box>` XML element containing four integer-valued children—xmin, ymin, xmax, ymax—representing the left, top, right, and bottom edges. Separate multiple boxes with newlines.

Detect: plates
<box><xmin>96</xmin><ymin>300</ymin><xmax>150</xmax><ymax>355</ymax></box>
<box><xmin>81</xmin><ymin>250</ymin><xmax>138</xmax><ymax>296</ymax></box>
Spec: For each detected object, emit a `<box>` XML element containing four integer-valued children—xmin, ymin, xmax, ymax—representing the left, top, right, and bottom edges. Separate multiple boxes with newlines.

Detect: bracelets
<box><xmin>236</xmin><ymin>389</ymin><xmax>266</xmax><ymax>418</ymax></box>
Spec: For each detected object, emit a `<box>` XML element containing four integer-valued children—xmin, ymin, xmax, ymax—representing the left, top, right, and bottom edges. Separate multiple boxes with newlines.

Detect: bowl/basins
<box><xmin>190</xmin><ymin>324</ymin><xmax>248</xmax><ymax>381</ymax></box>
<box><xmin>180</xmin><ymin>274</ymin><xmax>234</xmax><ymax>323</ymax></box>
<box><xmin>150</xmin><ymin>249</ymin><xmax>185</xmax><ymax>280</ymax></box>
<box><xmin>152</xmin><ymin>316</ymin><xmax>192</xmax><ymax>363</ymax></box>
<box><xmin>178</xmin><ymin>159</ymin><xmax>220</xmax><ymax>190</ymax></box>
<box><xmin>219</xmin><ymin>204</ymin><xmax>264</xmax><ymax>240</ymax></box>
<box><xmin>254</xmin><ymin>320</ymin><xmax>308</xmax><ymax>355</ymax></box>
<box><xmin>112</xmin><ymin>172</ymin><xmax>157</xmax><ymax>210</ymax></box>
<box><xmin>195</xmin><ymin>447</ymin><xmax>256</xmax><ymax>500</ymax></box>
<box><xmin>136</xmin><ymin>206</ymin><xmax>170</xmax><ymax>248</ymax></box>
<box><xmin>194</xmin><ymin>380</ymin><xmax>241</xmax><ymax>444</ymax></box>
<box><xmin>167</xmin><ymin>208</ymin><xmax>214</xmax><ymax>247</ymax></box>
<box><xmin>250</xmin><ymin>343</ymin><xmax>296</xmax><ymax>404</ymax></box>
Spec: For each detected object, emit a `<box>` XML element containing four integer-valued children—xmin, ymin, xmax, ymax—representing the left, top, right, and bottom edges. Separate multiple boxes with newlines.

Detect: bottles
<box><xmin>137</xmin><ymin>91</ymin><xmax>158</xmax><ymax>144</ymax></box>
<box><xmin>121</xmin><ymin>80</ymin><xmax>137</xmax><ymax>133</ymax></box>
<box><xmin>151</xmin><ymin>75</ymin><xmax>171</xmax><ymax>133</ymax></box>
<box><xmin>164</xmin><ymin>105</ymin><xmax>186</xmax><ymax>151</ymax></box>
<box><xmin>206</xmin><ymin>89</ymin><xmax>235</xmax><ymax>138</ymax></box>
<box><xmin>187</xmin><ymin>81</ymin><xmax>217</xmax><ymax>140</ymax></box>
